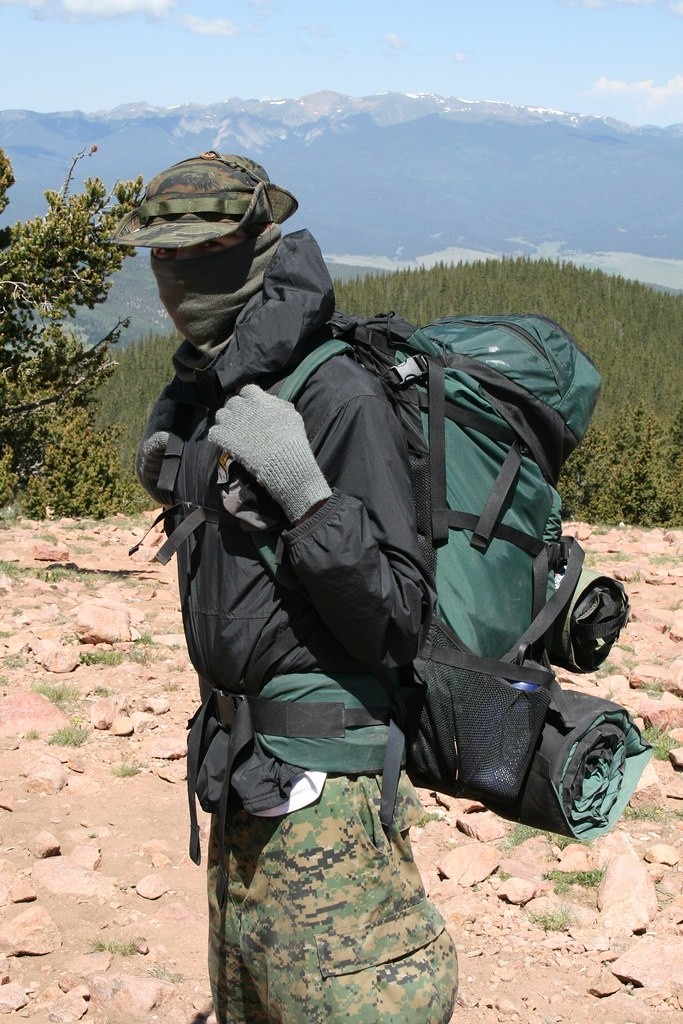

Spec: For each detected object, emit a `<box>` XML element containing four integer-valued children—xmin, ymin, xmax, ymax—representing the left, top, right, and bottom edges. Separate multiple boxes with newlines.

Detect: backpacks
<box><xmin>158</xmin><ymin>308</ymin><xmax>606</xmax><ymax>802</ymax></box>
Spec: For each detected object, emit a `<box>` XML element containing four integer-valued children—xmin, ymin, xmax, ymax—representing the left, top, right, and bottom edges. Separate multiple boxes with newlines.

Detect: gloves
<box><xmin>139</xmin><ymin>430</ymin><xmax>170</xmax><ymax>505</ymax></box>
<box><xmin>208</xmin><ymin>381</ymin><xmax>333</xmax><ymax>524</ymax></box>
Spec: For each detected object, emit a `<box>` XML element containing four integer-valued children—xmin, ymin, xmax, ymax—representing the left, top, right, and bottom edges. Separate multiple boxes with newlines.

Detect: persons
<box><xmin>107</xmin><ymin>151</ymin><xmax>458</xmax><ymax>1024</ymax></box>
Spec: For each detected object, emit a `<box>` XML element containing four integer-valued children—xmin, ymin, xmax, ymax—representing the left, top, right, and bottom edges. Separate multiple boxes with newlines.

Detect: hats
<box><xmin>109</xmin><ymin>149</ymin><xmax>300</xmax><ymax>249</ymax></box>
